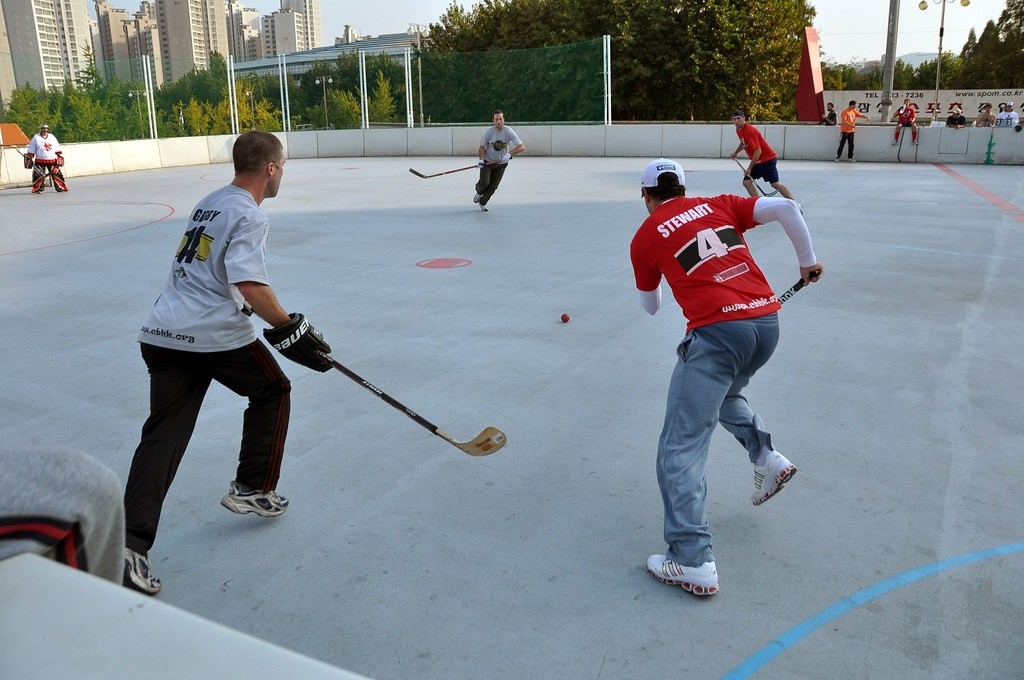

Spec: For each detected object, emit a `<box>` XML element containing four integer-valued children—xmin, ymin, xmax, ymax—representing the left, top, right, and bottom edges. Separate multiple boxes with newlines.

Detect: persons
<box><xmin>24</xmin><ymin>124</ymin><xmax>69</xmax><ymax>193</ymax></box>
<box><xmin>946</xmin><ymin>109</ymin><xmax>967</xmax><ymax>129</ymax></box>
<box><xmin>996</xmin><ymin>101</ymin><xmax>1020</xmax><ymax>127</ymax></box>
<box><xmin>0</xmin><ymin>445</ymin><xmax>123</xmax><ymax>584</ymax></box>
<box><xmin>891</xmin><ymin>99</ymin><xmax>919</xmax><ymax>145</ymax></box>
<box><xmin>730</xmin><ymin>109</ymin><xmax>805</xmax><ymax>218</ymax></box>
<box><xmin>973</xmin><ymin>103</ymin><xmax>996</xmax><ymax>127</ymax></box>
<box><xmin>822</xmin><ymin>102</ymin><xmax>837</xmax><ymax>126</ymax></box>
<box><xmin>473</xmin><ymin>110</ymin><xmax>525</xmax><ymax>212</ymax></box>
<box><xmin>118</xmin><ymin>131</ymin><xmax>334</xmax><ymax>593</ymax></box>
<box><xmin>628</xmin><ymin>158</ymin><xmax>824</xmax><ymax>598</ymax></box>
<box><xmin>835</xmin><ymin>100</ymin><xmax>870</xmax><ymax>164</ymax></box>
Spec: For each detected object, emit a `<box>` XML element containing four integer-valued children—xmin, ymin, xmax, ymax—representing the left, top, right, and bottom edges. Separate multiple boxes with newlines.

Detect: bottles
<box><xmin>900</xmin><ymin>104</ymin><xmax>906</xmax><ymax>114</ymax></box>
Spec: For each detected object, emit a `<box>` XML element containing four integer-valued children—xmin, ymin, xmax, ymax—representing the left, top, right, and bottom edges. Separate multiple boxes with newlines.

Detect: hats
<box><xmin>640</xmin><ymin>158</ymin><xmax>686</xmax><ymax>198</ymax></box>
<box><xmin>1007</xmin><ymin>102</ymin><xmax>1013</xmax><ymax>106</ymax></box>
<box><xmin>953</xmin><ymin>109</ymin><xmax>961</xmax><ymax>114</ymax></box>
<box><xmin>983</xmin><ymin>103</ymin><xmax>992</xmax><ymax>108</ymax></box>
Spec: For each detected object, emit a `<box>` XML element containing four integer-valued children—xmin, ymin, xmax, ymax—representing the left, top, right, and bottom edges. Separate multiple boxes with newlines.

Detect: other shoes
<box><xmin>848</xmin><ymin>159</ymin><xmax>856</xmax><ymax>162</ymax></box>
<box><xmin>912</xmin><ymin>141</ymin><xmax>918</xmax><ymax>145</ymax></box>
<box><xmin>892</xmin><ymin>141</ymin><xmax>900</xmax><ymax>144</ymax></box>
<box><xmin>835</xmin><ymin>159</ymin><xmax>839</xmax><ymax>161</ymax></box>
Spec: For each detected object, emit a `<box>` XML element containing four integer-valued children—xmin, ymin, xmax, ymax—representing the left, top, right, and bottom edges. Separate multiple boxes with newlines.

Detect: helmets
<box><xmin>39</xmin><ymin>124</ymin><xmax>49</xmax><ymax>128</ymax></box>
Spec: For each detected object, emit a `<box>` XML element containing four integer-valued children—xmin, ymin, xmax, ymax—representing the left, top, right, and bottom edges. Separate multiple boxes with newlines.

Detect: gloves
<box><xmin>502</xmin><ymin>153</ymin><xmax>511</xmax><ymax>163</ymax></box>
<box><xmin>478</xmin><ymin>160</ymin><xmax>484</xmax><ymax>167</ymax></box>
<box><xmin>263</xmin><ymin>313</ymin><xmax>334</xmax><ymax>373</ymax></box>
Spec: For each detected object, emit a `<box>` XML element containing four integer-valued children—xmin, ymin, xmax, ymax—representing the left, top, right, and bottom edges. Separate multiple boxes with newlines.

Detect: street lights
<box><xmin>919</xmin><ymin>0</ymin><xmax>972</xmax><ymax>121</ymax></box>
<box><xmin>246</xmin><ymin>91</ymin><xmax>254</xmax><ymax>131</ymax></box>
<box><xmin>316</xmin><ymin>76</ymin><xmax>333</xmax><ymax>130</ymax></box>
<box><xmin>129</xmin><ymin>90</ymin><xmax>147</xmax><ymax>138</ymax></box>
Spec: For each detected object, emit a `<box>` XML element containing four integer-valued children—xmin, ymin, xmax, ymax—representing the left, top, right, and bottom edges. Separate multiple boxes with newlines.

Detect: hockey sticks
<box><xmin>409</xmin><ymin>156</ymin><xmax>512</xmax><ymax>179</ymax></box>
<box><xmin>897</xmin><ymin>111</ymin><xmax>910</xmax><ymax>161</ymax></box>
<box><xmin>734</xmin><ymin>156</ymin><xmax>781</xmax><ymax>197</ymax></box>
<box><xmin>778</xmin><ymin>271</ymin><xmax>820</xmax><ymax>305</ymax></box>
<box><xmin>15</xmin><ymin>148</ymin><xmax>45</xmax><ymax>178</ymax></box>
<box><xmin>239</xmin><ymin>299</ymin><xmax>507</xmax><ymax>457</ymax></box>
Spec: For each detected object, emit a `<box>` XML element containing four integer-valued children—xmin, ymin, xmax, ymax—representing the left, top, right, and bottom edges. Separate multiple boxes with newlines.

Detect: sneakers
<box><xmin>478</xmin><ymin>203</ymin><xmax>489</xmax><ymax>211</ymax></box>
<box><xmin>220</xmin><ymin>480</ymin><xmax>288</xmax><ymax>517</ymax></box>
<box><xmin>473</xmin><ymin>193</ymin><xmax>481</xmax><ymax>203</ymax></box>
<box><xmin>751</xmin><ymin>450</ymin><xmax>797</xmax><ymax>505</ymax></box>
<box><xmin>124</xmin><ymin>548</ymin><xmax>160</xmax><ymax>592</ymax></box>
<box><xmin>646</xmin><ymin>554</ymin><xmax>720</xmax><ymax>595</ymax></box>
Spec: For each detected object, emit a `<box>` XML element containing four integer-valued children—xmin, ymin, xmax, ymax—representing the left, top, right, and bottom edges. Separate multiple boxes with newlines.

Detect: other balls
<box><xmin>561</xmin><ymin>314</ymin><xmax>569</xmax><ymax>323</ymax></box>
